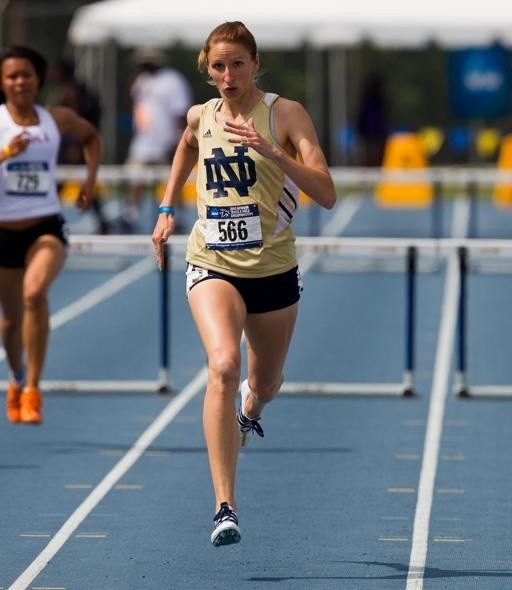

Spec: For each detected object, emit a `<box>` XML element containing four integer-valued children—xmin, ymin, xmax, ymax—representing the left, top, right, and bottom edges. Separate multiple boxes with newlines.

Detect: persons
<box><xmin>45</xmin><ymin>56</ymin><xmax>98</xmax><ymax>165</ymax></box>
<box><xmin>0</xmin><ymin>45</ymin><xmax>104</xmax><ymax>423</ymax></box>
<box><xmin>151</xmin><ymin>20</ymin><xmax>336</xmax><ymax>548</ymax></box>
<box><xmin>121</xmin><ymin>62</ymin><xmax>168</xmax><ymax>166</ymax></box>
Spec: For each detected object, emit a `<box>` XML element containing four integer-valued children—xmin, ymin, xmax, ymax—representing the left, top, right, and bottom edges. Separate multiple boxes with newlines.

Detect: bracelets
<box><xmin>158</xmin><ymin>206</ymin><xmax>176</xmax><ymax>215</ymax></box>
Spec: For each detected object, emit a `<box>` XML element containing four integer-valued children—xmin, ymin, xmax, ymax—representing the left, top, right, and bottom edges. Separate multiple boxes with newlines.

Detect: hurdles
<box><xmin>2</xmin><ymin>232</ymin><xmax>173</xmax><ymax>392</ymax></box>
<box><xmin>309</xmin><ymin>172</ymin><xmax>454</xmax><ymax>273</ymax></box>
<box><xmin>58</xmin><ymin>165</ymin><xmax>190</xmax><ymax>269</ymax></box>
<box><xmin>439</xmin><ymin>239</ymin><xmax>511</xmax><ymax>401</ymax></box>
<box><xmin>454</xmin><ymin>174</ymin><xmax>511</xmax><ymax>275</ymax></box>
<box><xmin>279</xmin><ymin>236</ymin><xmax>440</xmax><ymax>399</ymax></box>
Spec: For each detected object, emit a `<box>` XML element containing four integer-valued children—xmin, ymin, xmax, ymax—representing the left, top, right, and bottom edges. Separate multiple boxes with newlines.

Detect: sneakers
<box><xmin>7</xmin><ymin>378</ymin><xmax>42</xmax><ymax>422</ymax></box>
<box><xmin>238</xmin><ymin>378</ymin><xmax>261</xmax><ymax>446</ymax></box>
<box><xmin>211</xmin><ymin>501</ymin><xmax>242</xmax><ymax>547</ymax></box>
